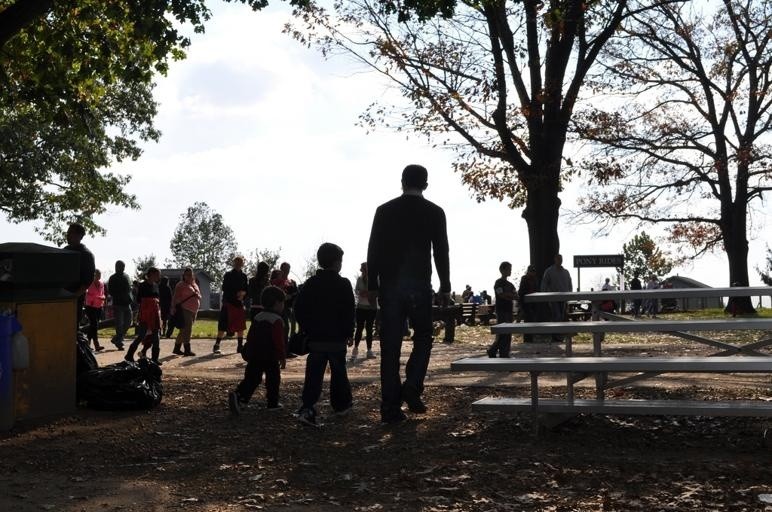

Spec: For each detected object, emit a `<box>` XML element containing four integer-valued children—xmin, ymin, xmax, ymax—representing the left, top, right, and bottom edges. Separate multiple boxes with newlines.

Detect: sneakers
<box><xmin>297</xmin><ymin>406</ymin><xmax>320</xmax><ymax>427</ymax></box>
<box><xmin>229</xmin><ymin>390</ymin><xmax>241</xmax><ymax>414</ymax></box>
<box><xmin>268</xmin><ymin>403</ymin><xmax>283</xmax><ymax>411</ymax></box>
<box><xmin>401</xmin><ymin>380</ymin><xmax>426</xmax><ymax>413</ymax></box>
<box><xmin>335</xmin><ymin>400</ymin><xmax>355</xmax><ymax>417</ymax></box>
<box><xmin>96</xmin><ymin>331</ymin><xmax>195</xmax><ymax>365</ymax></box>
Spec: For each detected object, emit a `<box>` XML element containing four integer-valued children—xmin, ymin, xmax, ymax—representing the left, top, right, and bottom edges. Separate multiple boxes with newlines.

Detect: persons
<box><xmin>367</xmin><ymin>165</ymin><xmax>451</xmax><ymax>416</ymax></box>
<box><xmin>294</xmin><ymin>242</ymin><xmax>354</xmax><ymax>424</ymax></box>
<box><xmin>228</xmin><ymin>285</ymin><xmax>290</xmax><ymax>411</ymax></box>
<box><xmin>645</xmin><ymin>274</ymin><xmax>659</xmax><ymax>317</ymax></box>
<box><xmin>487</xmin><ymin>262</ymin><xmax>520</xmax><ymax>358</ymax></box>
<box><xmin>631</xmin><ymin>271</ymin><xmax>642</xmax><ymax>317</ymax></box>
<box><xmin>125</xmin><ymin>243</ymin><xmax>355</xmax><ymax>364</ymax></box>
<box><xmin>85</xmin><ymin>270</ymin><xmax>105</xmax><ymax>352</ymax></box>
<box><xmin>108</xmin><ymin>260</ymin><xmax>134</xmax><ymax>350</ymax></box>
<box><xmin>352</xmin><ymin>262</ymin><xmax>380</xmax><ymax>358</ymax></box>
<box><xmin>602</xmin><ymin>279</ymin><xmax>610</xmax><ymax>290</ymax></box>
<box><xmin>461</xmin><ymin>286</ymin><xmax>492</xmax><ymax>326</ymax></box>
<box><xmin>62</xmin><ymin>224</ymin><xmax>94</xmax><ymax>328</ymax></box>
<box><xmin>518</xmin><ymin>265</ymin><xmax>539</xmax><ymax>342</ymax></box>
<box><xmin>541</xmin><ymin>254</ymin><xmax>572</xmax><ymax>341</ymax></box>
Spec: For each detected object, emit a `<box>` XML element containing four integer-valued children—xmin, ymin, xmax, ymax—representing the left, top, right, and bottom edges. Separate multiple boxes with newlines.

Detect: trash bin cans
<box><xmin>0</xmin><ymin>311</ymin><xmax>24</xmax><ymax>433</ymax></box>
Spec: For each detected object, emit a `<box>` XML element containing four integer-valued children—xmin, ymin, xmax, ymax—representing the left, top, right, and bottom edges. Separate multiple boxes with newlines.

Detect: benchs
<box><xmin>449</xmin><ymin>286</ymin><xmax>772</xmax><ymax>418</ymax></box>
